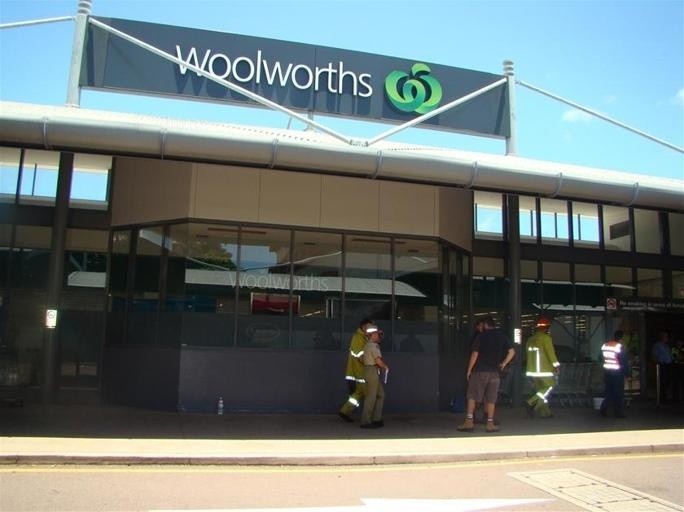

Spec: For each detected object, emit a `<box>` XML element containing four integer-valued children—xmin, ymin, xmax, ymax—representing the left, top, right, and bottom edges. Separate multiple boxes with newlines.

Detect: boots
<box><xmin>458</xmin><ymin>418</ymin><xmax>473</xmax><ymax>432</ymax></box>
<box><xmin>486</xmin><ymin>421</ymin><xmax>503</xmax><ymax>431</ymax></box>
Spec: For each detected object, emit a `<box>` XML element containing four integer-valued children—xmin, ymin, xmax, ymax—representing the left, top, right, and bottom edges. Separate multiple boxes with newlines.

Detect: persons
<box><xmin>600</xmin><ymin>330</ymin><xmax>630</xmax><ymax>418</ymax></box>
<box><xmin>457</xmin><ymin>315</ymin><xmax>516</xmax><ymax>432</ymax></box>
<box><xmin>672</xmin><ymin>337</ymin><xmax>684</xmax><ymax>405</ymax></box>
<box><xmin>469</xmin><ymin>320</ymin><xmax>501</xmax><ymax>425</ymax></box>
<box><xmin>339</xmin><ymin>318</ymin><xmax>372</xmax><ymax>423</ymax></box>
<box><xmin>360</xmin><ymin>326</ymin><xmax>389</xmax><ymax>428</ymax></box>
<box><xmin>652</xmin><ymin>332</ymin><xmax>674</xmax><ymax>405</ymax></box>
<box><xmin>524</xmin><ymin>317</ymin><xmax>561</xmax><ymax>418</ymax></box>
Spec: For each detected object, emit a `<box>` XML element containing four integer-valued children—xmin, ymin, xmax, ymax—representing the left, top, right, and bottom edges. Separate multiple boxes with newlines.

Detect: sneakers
<box><xmin>360</xmin><ymin>421</ymin><xmax>384</xmax><ymax>428</ymax></box>
<box><xmin>338</xmin><ymin>412</ymin><xmax>354</xmax><ymax>422</ymax></box>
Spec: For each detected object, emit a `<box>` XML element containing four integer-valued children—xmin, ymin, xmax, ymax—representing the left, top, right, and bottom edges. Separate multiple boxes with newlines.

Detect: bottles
<box><xmin>216</xmin><ymin>396</ymin><xmax>224</xmax><ymax>415</ymax></box>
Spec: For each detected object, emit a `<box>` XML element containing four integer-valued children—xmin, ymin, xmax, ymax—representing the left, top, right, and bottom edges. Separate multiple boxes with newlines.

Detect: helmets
<box><xmin>536</xmin><ymin>319</ymin><xmax>551</xmax><ymax>327</ymax></box>
<box><xmin>364</xmin><ymin>326</ymin><xmax>383</xmax><ymax>335</ymax></box>
